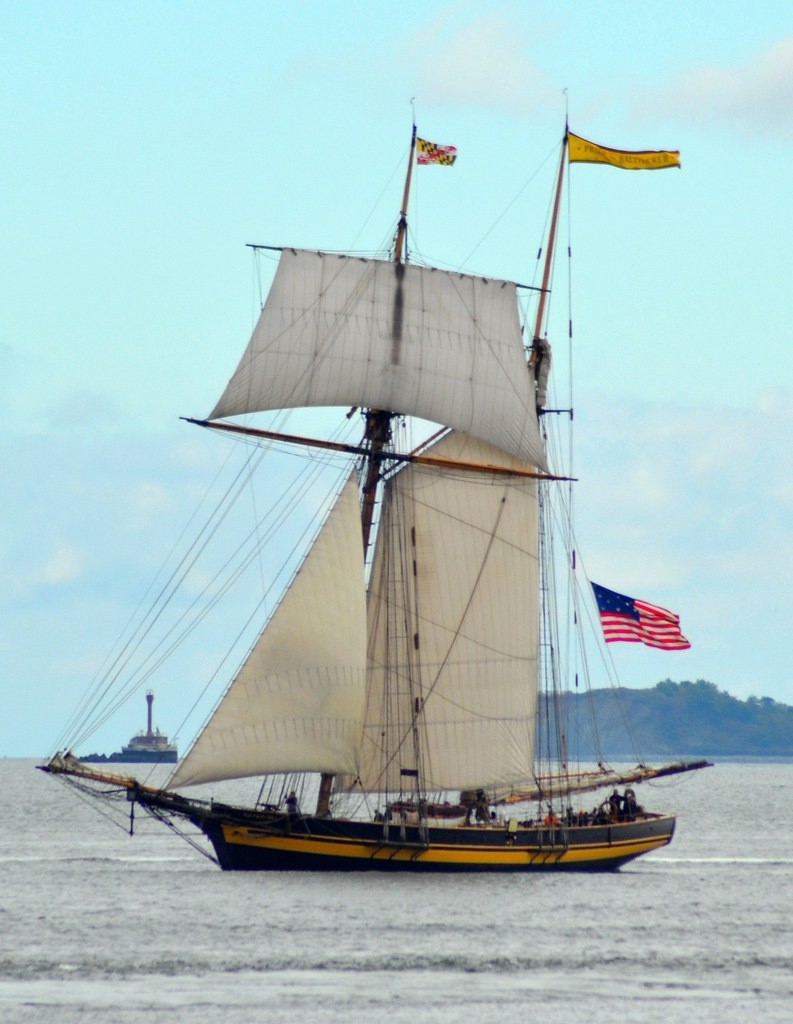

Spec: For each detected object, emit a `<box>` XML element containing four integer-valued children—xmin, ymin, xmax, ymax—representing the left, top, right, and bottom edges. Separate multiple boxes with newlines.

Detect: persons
<box><xmin>545</xmin><ymin>806</ymin><xmax>606</xmax><ymax>827</ymax></box>
<box><xmin>285</xmin><ymin>791</ymin><xmax>297</xmax><ymax>812</ymax></box>
<box><xmin>374</xmin><ymin>790</ymin><xmax>498</xmax><ymax>826</ymax></box>
<box><xmin>610</xmin><ymin>789</ymin><xmax>636</xmax><ymax>822</ymax></box>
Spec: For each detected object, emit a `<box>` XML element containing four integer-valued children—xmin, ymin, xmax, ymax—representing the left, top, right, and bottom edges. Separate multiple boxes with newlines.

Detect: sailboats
<box><xmin>34</xmin><ymin>85</ymin><xmax>715</xmax><ymax>872</ymax></box>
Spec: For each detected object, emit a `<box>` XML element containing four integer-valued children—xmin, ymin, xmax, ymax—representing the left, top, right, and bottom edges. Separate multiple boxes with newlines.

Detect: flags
<box><xmin>567</xmin><ymin>130</ymin><xmax>681</xmax><ymax>170</ymax></box>
<box><xmin>416</xmin><ymin>137</ymin><xmax>458</xmax><ymax>166</ymax></box>
<box><xmin>587</xmin><ymin>580</ymin><xmax>691</xmax><ymax>650</ymax></box>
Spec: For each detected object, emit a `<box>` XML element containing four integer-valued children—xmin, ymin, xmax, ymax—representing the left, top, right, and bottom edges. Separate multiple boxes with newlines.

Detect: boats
<box><xmin>77</xmin><ymin>691</ymin><xmax>178</xmax><ymax>763</ymax></box>
